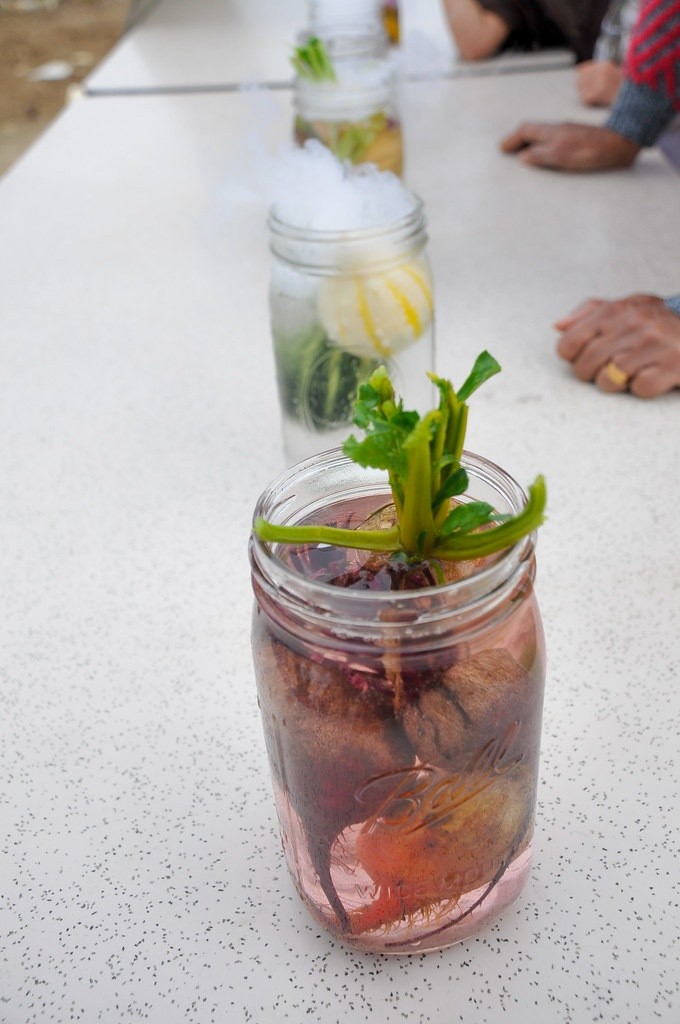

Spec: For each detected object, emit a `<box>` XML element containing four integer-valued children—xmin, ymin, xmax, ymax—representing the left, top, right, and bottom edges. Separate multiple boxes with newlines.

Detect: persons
<box><xmin>443</xmin><ymin>1</ymin><xmax>680</xmax><ymax>170</ymax></box>
<box><xmin>501</xmin><ymin>0</ymin><xmax>680</xmax><ymax>399</ymax></box>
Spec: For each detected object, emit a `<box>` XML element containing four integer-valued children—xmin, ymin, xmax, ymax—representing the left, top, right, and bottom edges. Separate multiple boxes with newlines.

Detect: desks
<box><xmin>0</xmin><ymin>66</ymin><xmax>680</xmax><ymax>1024</ymax></box>
<box><xmin>81</xmin><ymin>0</ymin><xmax>576</xmax><ymax>95</ymax></box>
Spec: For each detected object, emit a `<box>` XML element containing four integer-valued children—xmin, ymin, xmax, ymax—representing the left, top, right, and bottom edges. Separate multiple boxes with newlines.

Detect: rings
<box><xmin>606</xmin><ymin>361</ymin><xmax>629</xmax><ymax>385</ymax></box>
